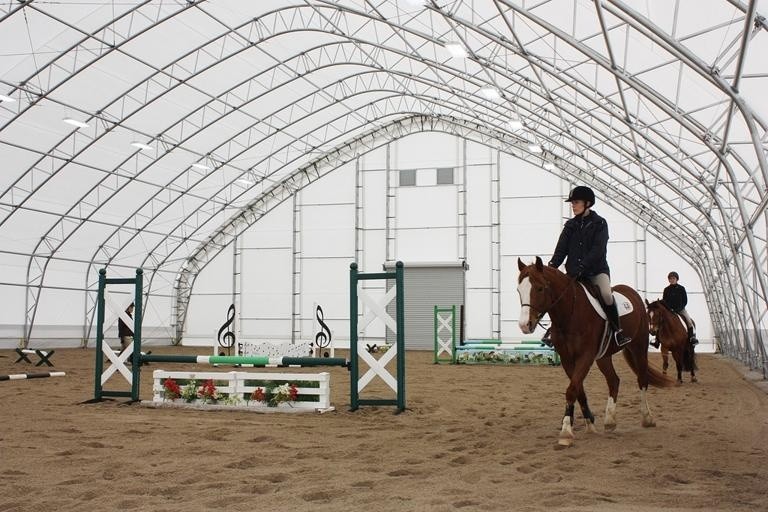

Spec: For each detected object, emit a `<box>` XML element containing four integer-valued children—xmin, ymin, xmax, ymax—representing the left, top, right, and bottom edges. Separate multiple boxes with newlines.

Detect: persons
<box><xmin>541</xmin><ymin>186</ymin><xmax>632</xmax><ymax>348</ymax></box>
<box><xmin>117</xmin><ymin>301</ymin><xmax>136</xmax><ymax>368</ymax></box>
<box><xmin>650</xmin><ymin>270</ymin><xmax>699</xmax><ymax>349</ymax></box>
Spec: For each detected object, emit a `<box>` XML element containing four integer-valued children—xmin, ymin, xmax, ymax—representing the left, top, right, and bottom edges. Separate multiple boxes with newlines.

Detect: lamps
<box><xmin>130</xmin><ymin>141</ymin><xmax>154</xmax><ymax>150</ymax></box>
<box><xmin>0</xmin><ymin>94</ymin><xmax>16</xmax><ymax>103</ymax></box>
<box><xmin>480</xmin><ymin>87</ymin><xmax>501</xmax><ymax>98</ymax></box>
<box><xmin>445</xmin><ymin>41</ymin><xmax>468</xmax><ymax>58</ymax></box>
<box><xmin>63</xmin><ymin>117</ymin><xmax>89</xmax><ymax>127</ymax></box>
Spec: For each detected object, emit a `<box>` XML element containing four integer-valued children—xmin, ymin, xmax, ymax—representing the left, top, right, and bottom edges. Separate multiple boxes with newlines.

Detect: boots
<box><xmin>607</xmin><ymin>295</ymin><xmax>630</xmax><ymax>347</ymax></box>
<box><xmin>650</xmin><ymin>340</ymin><xmax>659</xmax><ymax>349</ymax></box>
<box><xmin>688</xmin><ymin>326</ymin><xmax>698</xmax><ymax>346</ymax></box>
<box><xmin>541</xmin><ymin>336</ymin><xmax>551</xmax><ymax>343</ymax></box>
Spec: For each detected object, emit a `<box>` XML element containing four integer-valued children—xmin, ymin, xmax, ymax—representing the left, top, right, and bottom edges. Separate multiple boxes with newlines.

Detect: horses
<box><xmin>517</xmin><ymin>256</ymin><xmax>682</xmax><ymax>446</ymax></box>
<box><xmin>644</xmin><ymin>298</ymin><xmax>697</xmax><ymax>384</ymax></box>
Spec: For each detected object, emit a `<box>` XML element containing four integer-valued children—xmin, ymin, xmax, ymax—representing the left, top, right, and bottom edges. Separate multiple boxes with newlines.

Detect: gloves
<box><xmin>120</xmin><ymin>336</ymin><xmax>124</xmax><ymax>342</ymax></box>
<box><xmin>572</xmin><ymin>265</ymin><xmax>584</xmax><ymax>277</ymax></box>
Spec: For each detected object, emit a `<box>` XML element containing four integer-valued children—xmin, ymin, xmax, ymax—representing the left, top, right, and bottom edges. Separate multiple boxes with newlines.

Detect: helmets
<box><xmin>668</xmin><ymin>272</ymin><xmax>679</xmax><ymax>279</ymax></box>
<box><xmin>565</xmin><ymin>186</ymin><xmax>594</xmax><ymax>205</ymax></box>
